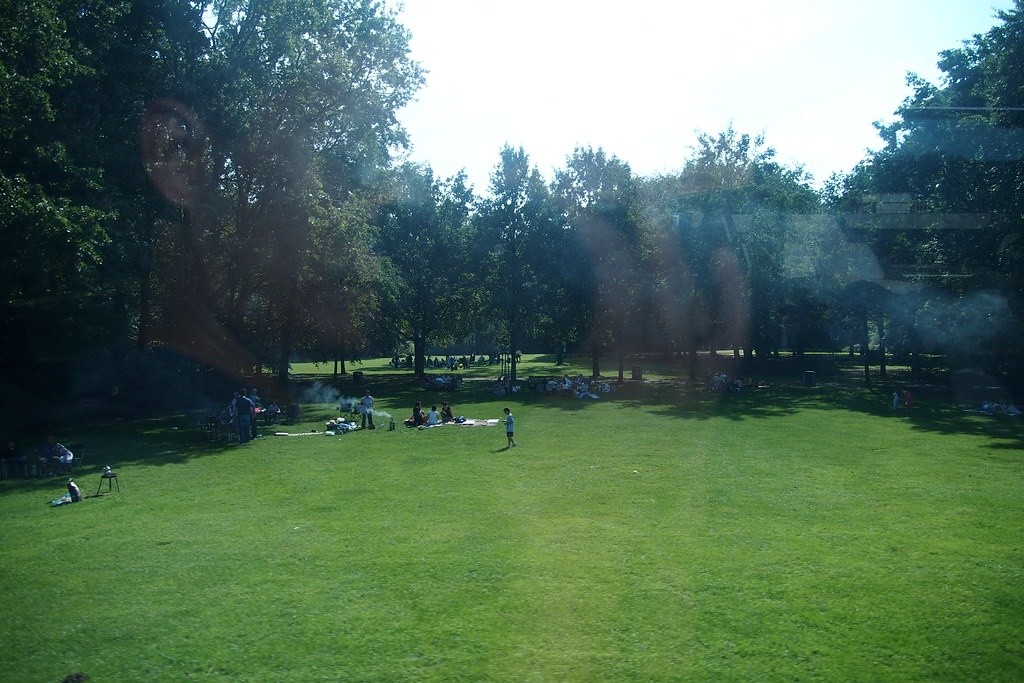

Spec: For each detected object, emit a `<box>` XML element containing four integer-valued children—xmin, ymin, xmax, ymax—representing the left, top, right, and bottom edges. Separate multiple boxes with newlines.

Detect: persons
<box><xmin>516</xmin><ymin>348</ymin><xmax>522</xmax><ymax>361</ymax></box>
<box><xmin>230</xmin><ymin>388</ymin><xmax>260</xmax><ymax>444</ymax></box>
<box><xmin>361</xmin><ymin>390</ymin><xmax>375</xmax><ymax>429</ymax></box>
<box><xmin>425</xmin><ymin>352</ymin><xmax>475</xmax><ymax>371</ymax></box>
<box><xmin>392</xmin><ymin>355</ymin><xmax>412</xmax><ymax>368</ymax></box>
<box><xmin>408</xmin><ymin>401</ymin><xmax>453</xmax><ymax>426</ymax></box>
<box><xmin>478</xmin><ymin>350</ymin><xmax>500</xmax><ymax>366</ymax></box>
<box><xmin>892</xmin><ymin>389</ymin><xmax>911</xmax><ymax>410</ymax></box>
<box><xmin>423</xmin><ymin>373</ymin><xmax>462</xmax><ymax>390</ymax></box>
<box><xmin>704</xmin><ymin>369</ymin><xmax>770</xmax><ymax>392</ymax></box>
<box><xmin>502</xmin><ymin>408</ymin><xmax>516</xmax><ymax>449</ymax></box>
<box><xmin>0</xmin><ymin>434</ymin><xmax>73</xmax><ymax>480</ymax></box>
<box><xmin>497</xmin><ymin>374</ymin><xmax>511</xmax><ymax>399</ymax></box>
<box><xmin>536</xmin><ymin>373</ymin><xmax>610</xmax><ymax>399</ymax></box>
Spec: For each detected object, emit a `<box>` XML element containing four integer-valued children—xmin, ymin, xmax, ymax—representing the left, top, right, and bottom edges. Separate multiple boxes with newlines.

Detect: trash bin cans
<box><xmin>631</xmin><ymin>366</ymin><xmax>642</xmax><ymax>380</ymax></box>
<box><xmin>353</xmin><ymin>372</ymin><xmax>363</xmax><ymax>386</ymax></box>
<box><xmin>803</xmin><ymin>370</ymin><xmax>816</xmax><ymax>387</ymax></box>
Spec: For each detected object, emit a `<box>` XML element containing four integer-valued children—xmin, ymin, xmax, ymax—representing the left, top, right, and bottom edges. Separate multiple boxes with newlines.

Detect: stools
<box><xmin>97</xmin><ymin>473</ymin><xmax>119</xmax><ymax>495</ymax></box>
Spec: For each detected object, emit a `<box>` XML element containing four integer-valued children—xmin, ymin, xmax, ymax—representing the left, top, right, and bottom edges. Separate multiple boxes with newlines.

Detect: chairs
<box><xmin>340</xmin><ymin>403</ymin><xmax>352</xmax><ymax>417</ymax></box>
<box><xmin>67</xmin><ymin>443</ymin><xmax>86</xmax><ymax>475</ymax></box>
<box><xmin>350</xmin><ymin>403</ymin><xmax>364</xmax><ymax>421</ymax></box>
